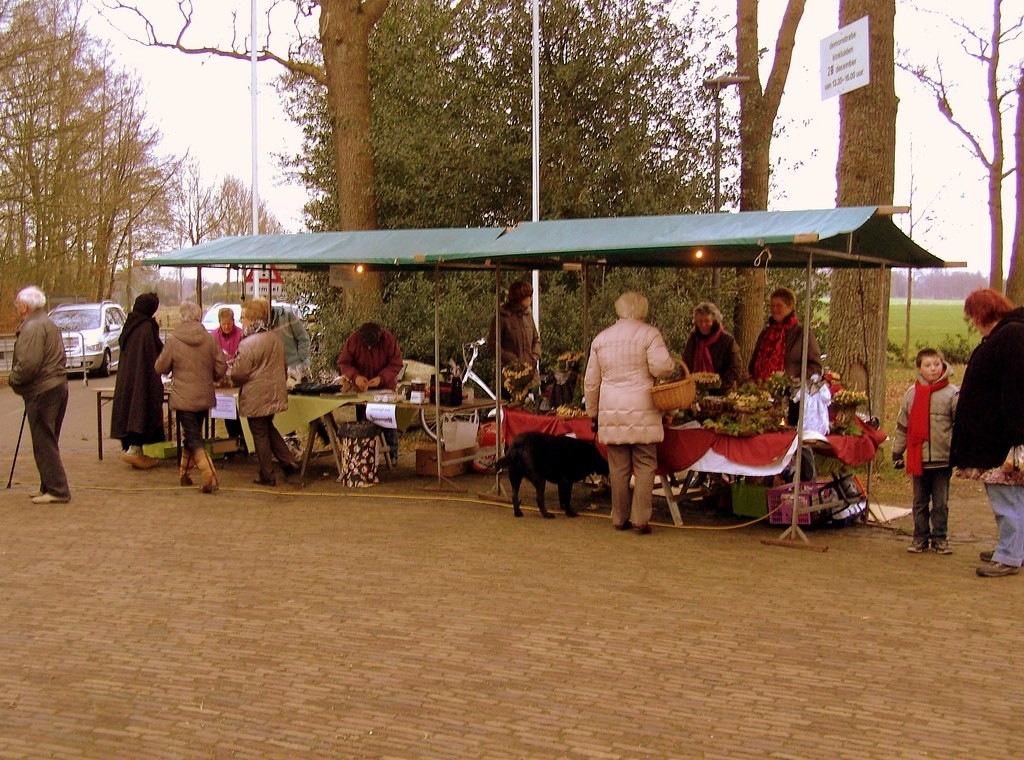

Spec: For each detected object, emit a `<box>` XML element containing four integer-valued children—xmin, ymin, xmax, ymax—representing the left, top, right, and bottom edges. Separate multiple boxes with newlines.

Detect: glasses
<box><xmin>240</xmin><ymin>316</ymin><xmax>246</xmax><ymax>320</ymax></box>
<box><xmin>14</xmin><ymin>301</ymin><xmax>22</xmax><ymax>307</ymax></box>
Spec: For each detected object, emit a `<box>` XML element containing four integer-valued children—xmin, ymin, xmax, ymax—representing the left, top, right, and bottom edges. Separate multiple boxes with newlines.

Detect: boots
<box><xmin>191</xmin><ymin>445</ymin><xmax>218</xmax><ymax>492</ymax></box>
<box><xmin>179</xmin><ymin>448</ymin><xmax>193</xmax><ymax>486</ymax></box>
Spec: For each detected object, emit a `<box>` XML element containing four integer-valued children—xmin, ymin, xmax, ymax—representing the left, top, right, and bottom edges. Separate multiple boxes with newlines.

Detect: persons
<box><xmin>153</xmin><ymin>301</ymin><xmax>228</xmax><ymax>493</ymax></box>
<box><xmin>948</xmin><ymin>289</ymin><xmax>1024</xmax><ymax>578</ymax></box>
<box><xmin>680</xmin><ymin>302</ymin><xmax>744</xmax><ymax>393</ymax></box>
<box><xmin>339</xmin><ymin>322</ymin><xmax>403</xmax><ymax>465</ymax></box>
<box><xmin>211</xmin><ymin>308</ymin><xmax>242</xmax><ymax>459</ymax></box>
<box><xmin>8</xmin><ymin>287</ymin><xmax>71</xmax><ymax>504</ymax></box>
<box><xmin>488</xmin><ymin>280</ymin><xmax>542</xmax><ymax>402</ymax></box>
<box><xmin>111</xmin><ymin>292</ymin><xmax>165</xmax><ymax>469</ymax></box>
<box><xmin>747</xmin><ymin>287</ymin><xmax>821</xmax><ymax>382</ymax></box>
<box><xmin>892</xmin><ymin>348</ymin><xmax>960</xmax><ymax>553</ymax></box>
<box><xmin>269</xmin><ymin>305</ymin><xmax>312</xmax><ymax>386</ymax></box>
<box><xmin>583</xmin><ymin>290</ymin><xmax>675</xmax><ymax>534</ymax></box>
<box><xmin>231</xmin><ymin>298</ymin><xmax>300</xmax><ymax>486</ymax></box>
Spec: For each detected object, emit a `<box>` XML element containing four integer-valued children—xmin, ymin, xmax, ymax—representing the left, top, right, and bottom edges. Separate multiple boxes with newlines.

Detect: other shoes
<box><xmin>122</xmin><ymin>454</ymin><xmax>159</xmax><ymax>469</ymax></box>
<box><xmin>254</xmin><ymin>479</ymin><xmax>276</xmax><ymax>486</ymax></box>
<box><xmin>283</xmin><ymin>462</ymin><xmax>298</xmax><ymax>483</ymax></box>
<box><xmin>29</xmin><ymin>489</ymin><xmax>71</xmax><ymax>503</ymax></box>
<box><xmin>391</xmin><ymin>458</ymin><xmax>398</xmax><ymax>466</ymax></box>
<box><xmin>613</xmin><ymin>520</ymin><xmax>633</xmax><ymax>531</ymax></box>
<box><xmin>633</xmin><ymin>524</ymin><xmax>652</xmax><ymax>534</ymax></box>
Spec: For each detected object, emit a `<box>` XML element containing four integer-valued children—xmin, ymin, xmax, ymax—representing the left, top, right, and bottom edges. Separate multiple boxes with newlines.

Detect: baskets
<box><xmin>650</xmin><ymin>360</ymin><xmax>696</xmax><ymax>413</ymax></box>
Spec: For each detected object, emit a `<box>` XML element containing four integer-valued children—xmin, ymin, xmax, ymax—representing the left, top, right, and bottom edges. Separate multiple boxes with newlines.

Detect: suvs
<box><xmin>201</xmin><ymin>302</ymin><xmax>242</xmax><ymax>334</ymax></box>
<box><xmin>47</xmin><ymin>299</ymin><xmax>126</xmax><ymax>377</ymax></box>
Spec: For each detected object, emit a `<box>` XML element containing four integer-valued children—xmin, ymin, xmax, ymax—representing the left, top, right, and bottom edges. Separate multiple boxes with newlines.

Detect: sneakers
<box><xmin>980</xmin><ymin>549</ymin><xmax>1024</xmax><ymax>567</ymax></box>
<box><xmin>907</xmin><ymin>536</ymin><xmax>953</xmax><ymax>554</ymax></box>
<box><xmin>975</xmin><ymin>562</ymin><xmax>1019</xmax><ymax>576</ymax></box>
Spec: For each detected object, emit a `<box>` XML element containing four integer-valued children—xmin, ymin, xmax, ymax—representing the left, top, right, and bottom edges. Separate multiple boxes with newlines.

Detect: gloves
<box><xmin>591</xmin><ymin>416</ymin><xmax>599</xmax><ymax>433</ymax></box>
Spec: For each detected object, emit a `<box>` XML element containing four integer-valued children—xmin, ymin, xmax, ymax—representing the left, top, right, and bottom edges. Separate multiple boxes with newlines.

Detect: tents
<box><xmin>131</xmin><ymin>206</ymin><xmax>967</xmax><ymax>552</ymax></box>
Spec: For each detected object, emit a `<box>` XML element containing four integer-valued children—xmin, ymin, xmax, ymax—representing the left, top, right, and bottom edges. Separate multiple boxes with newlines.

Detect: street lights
<box><xmin>705</xmin><ymin>74</ymin><xmax>751</xmax><ymax>296</ymax></box>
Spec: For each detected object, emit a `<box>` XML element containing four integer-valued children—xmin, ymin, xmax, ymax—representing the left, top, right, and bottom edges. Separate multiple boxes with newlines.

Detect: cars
<box><xmin>272</xmin><ymin>301</ymin><xmax>318</xmax><ymax>318</ymax></box>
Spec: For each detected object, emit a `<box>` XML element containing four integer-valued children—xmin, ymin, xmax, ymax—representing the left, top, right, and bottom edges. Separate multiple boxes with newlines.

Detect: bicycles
<box><xmin>419</xmin><ymin>336</ymin><xmax>505</xmax><ymax>444</ymax></box>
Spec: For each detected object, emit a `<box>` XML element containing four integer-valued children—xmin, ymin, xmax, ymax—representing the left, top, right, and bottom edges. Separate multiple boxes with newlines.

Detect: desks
<box><xmin>502</xmin><ymin>409</ymin><xmax>886</xmax><ymax>528</ymax></box>
<box><xmin>92</xmin><ymin>386</ymin><xmax>510</xmax><ymax>486</ymax></box>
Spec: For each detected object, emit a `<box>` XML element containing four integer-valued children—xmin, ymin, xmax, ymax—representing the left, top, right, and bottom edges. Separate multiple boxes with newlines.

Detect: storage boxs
<box><xmin>768</xmin><ymin>479</ymin><xmax>830</xmax><ymax>525</ymax></box>
<box><xmin>210</xmin><ymin>437</ymin><xmax>238</xmax><ymax>454</ymax></box>
<box><xmin>415</xmin><ymin>445</ymin><xmax>466</xmax><ymax>476</ymax></box>
<box><xmin>141</xmin><ymin>440</ymin><xmax>181</xmax><ymax>458</ymax></box>
<box><xmin>731</xmin><ymin>480</ymin><xmax>769</xmax><ymax>519</ymax></box>
<box><xmin>204</xmin><ymin>439</ymin><xmax>224</xmax><ymax>460</ymax></box>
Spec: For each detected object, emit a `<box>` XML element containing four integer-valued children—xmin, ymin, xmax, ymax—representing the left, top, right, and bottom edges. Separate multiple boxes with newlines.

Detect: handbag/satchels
<box><xmin>430</xmin><ymin>374</ymin><xmax>462</xmax><ymax>407</ymax></box>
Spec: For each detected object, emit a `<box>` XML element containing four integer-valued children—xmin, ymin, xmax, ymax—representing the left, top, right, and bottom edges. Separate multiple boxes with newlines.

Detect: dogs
<box><xmin>494</xmin><ymin>430</ymin><xmax>610</xmax><ymax>519</ymax></box>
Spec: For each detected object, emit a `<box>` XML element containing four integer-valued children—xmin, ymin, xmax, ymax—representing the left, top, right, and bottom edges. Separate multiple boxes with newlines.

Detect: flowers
<box><xmin>502</xmin><ymin>351</ymin><xmax>585</xmax><ymax>401</ymax></box>
<box><xmin>687</xmin><ymin>369</ymin><xmax>868</xmax><ymax>410</ymax></box>
